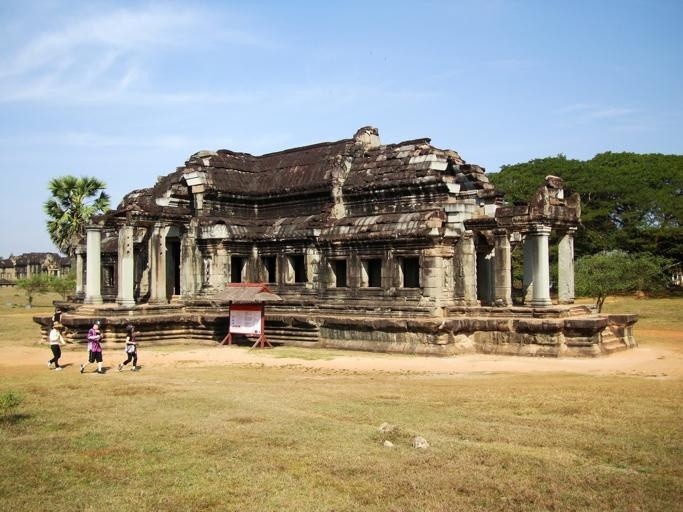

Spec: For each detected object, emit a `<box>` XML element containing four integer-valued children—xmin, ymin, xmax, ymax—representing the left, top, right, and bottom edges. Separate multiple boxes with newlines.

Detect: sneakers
<box><xmin>118</xmin><ymin>364</ymin><xmax>122</xmax><ymax>371</ymax></box>
<box><xmin>80</xmin><ymin>364</ymin><xmax>84</xmax><ymax>374</ymax></box>
<box><xmin>47</xmin><ymin>360</ymin><xmax>52</xmax><ymax>369</ymax></box>
<box><xmin>54</xmin><ymin>367</ymin><xmax>62</xmax><ymax>371</ymax></box>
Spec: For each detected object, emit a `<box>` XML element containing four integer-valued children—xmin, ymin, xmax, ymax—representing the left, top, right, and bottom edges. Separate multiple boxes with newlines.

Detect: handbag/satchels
<box><xmin>124</xmin><ymin>344</ymin><xmax>136</xmax><ymax>353</ymax></box>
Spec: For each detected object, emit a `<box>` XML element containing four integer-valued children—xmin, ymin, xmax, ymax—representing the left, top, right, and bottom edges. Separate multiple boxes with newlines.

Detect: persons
<box><xmin>45</xmin><ymin>324</ymin><xmax>66</xmax><ymax>371</ymax></box>
<box><xmin>50</xmin><ymin>307</ymin><xmax>67</xmax><ymax>343</ymax></box>
<box><xmin>77</xmin><ymin>319</ymin><xmax>103</xmax><ymax>375</ymax></box>
<box><xmin>114</xmin><ymin>324</ymin><xmax>143</xmax><ymax>374</ymax></box>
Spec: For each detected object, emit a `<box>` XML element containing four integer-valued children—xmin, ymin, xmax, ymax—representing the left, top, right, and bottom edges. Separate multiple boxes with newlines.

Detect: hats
<box><xmin>53</xmin><ymin>321</ymin><xmax>63</xmax><ymax>327</ymax></box>
